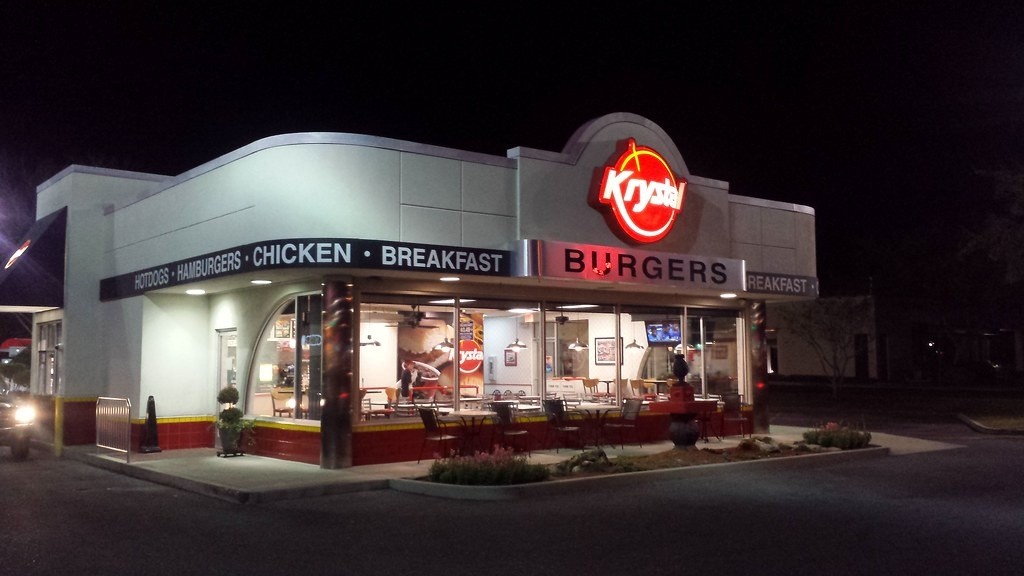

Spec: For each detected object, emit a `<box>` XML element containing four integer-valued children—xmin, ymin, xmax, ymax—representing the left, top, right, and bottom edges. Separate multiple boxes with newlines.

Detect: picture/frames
<box><xmin>504</xmin><ymin>350</ymin><xmax>517</xmax><ymax>366</ymax></box>
<box><xmin>595</xmin><ymin>337</ymin><xmax>624</xmax><ymax>365</ymax></box>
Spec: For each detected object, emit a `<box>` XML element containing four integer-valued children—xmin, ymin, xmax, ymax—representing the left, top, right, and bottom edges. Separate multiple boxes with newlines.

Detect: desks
<box><xmin>433</xmin><ymin>408</ymin><xmax>455</xmax><ymax>416</ymax></box>
<box><xmin>520</xmin><ymin>396</ymin><xmax>541</xmax><ymax>403</ymax></box>
<box><xmin>643</xmin><ymin>381</ymin><xmax>668</xmax><ymax>397</ymax></box>
<box><xmin>510</xmin><ymin>405</ymin><xmax>542</xmax><ymax>413</ymax></box>
<box><xmin>450</xmin><ymin>408</ymin><xmax>498</xmax><ymax>453</ymax></box>
<box><xmin>459</xmin><ymin>398</ymin><xmax>483</xmax><ymax>409</ymax></box>
<box><xmin>364</xmin><ymin>390</ymin><xmax>382</xmax><ymax>393</ymax></box>
<box><xmin>575</xmin><ymin>406</ymin><xmax>620</xmax><ymax>449</ymax></box>
<box><xmin>597</xmin><ymin>380</ymin><xmax>614</xmax><ymax>396</ymax></box>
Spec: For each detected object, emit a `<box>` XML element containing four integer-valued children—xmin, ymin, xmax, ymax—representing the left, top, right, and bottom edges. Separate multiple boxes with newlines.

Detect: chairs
<box><xmin>418</xmin><ymin>408</ymin><xmax>458</xmax><ymax>464</ymax></box>
<box><xmin>487</xmin><ymin>403</ymin><xmax>532</xmax><ymax>458</ymax></box>
<box><xmin>540</xmin><ymin>399</ymin><xmax>585</xmax><ymax>454</ymax></box>
<box><xmin>719</xmin><ymin>393</ymin><xmax>752</xmax><ymax>439</ymax></box>
<box><xmin>270</xmin><ymin>389</ymin><xmax>309</xmax><ymax>418</ymax></box>
<box><xmin>359</xmin><ymin>387</ymin><xmax>584</xmax><ymax>419</ymax></box>
<box><xmin>692</xmin><ymin>400</ymin><xmax>720</xmax><ymax>444</ymax></box>
<box><xmin>608</xmin><ymin>398</ymin><xmax>644</xmax><ymax>449</ymax></box>
<box><xmin>585</xmin><ymin>378</ymin><xmax>692</xmax><ymax>406</ymax></box>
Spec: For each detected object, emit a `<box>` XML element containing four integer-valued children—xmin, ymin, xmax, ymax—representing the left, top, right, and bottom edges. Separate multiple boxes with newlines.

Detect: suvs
<box><xmin>0</xmin><ymin>390</ymin><xmax>36</xmax><ymax>460</ymax></box>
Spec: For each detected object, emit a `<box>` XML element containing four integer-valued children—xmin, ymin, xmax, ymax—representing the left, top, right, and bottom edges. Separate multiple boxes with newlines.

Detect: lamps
<box><xmin>568</xmin><ymin>312</ymin><xmax>589</xmax><ymax>349</ymax></box>
<box><xmin>506</xmin><ymin>313</ymin><xmax>527</xmax><ymax>352</ymax></box>
<box><xmin>626</xmin><ymin>324</ymin><xmax>644</xmax><ymax>349</ymax></box>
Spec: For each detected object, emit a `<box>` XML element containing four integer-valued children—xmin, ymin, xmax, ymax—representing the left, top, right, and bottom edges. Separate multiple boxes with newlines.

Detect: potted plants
<box><xmin>209</xmin><ymin>387</ymin><xmax>256</xmax><ymax>457</ymax></box>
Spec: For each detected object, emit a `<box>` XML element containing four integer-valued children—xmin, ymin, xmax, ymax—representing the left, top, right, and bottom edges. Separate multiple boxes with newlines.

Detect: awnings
<box><xmin>0</xmin><ymin>205</ymin><xmax>68</xmax><ymax>312</ymax></box>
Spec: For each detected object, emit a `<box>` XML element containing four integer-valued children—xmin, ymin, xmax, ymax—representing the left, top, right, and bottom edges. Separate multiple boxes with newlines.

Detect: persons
<box><xmin>670</xmin><ymin>323</ymin><xmax>681</xmax><ymax>339</ymax></box>
<box><xmin>648</xmin><ymin>326</ymin><xmax>660</xmax><ymax>341</ymax></box>
<box><xmin>401</xmin><ymin>359</ymin><xmax>414</xmax><ymax>397</ymax></box>
<box><xmin>658</xmin><ymin>323</ymin><xmax>671</xmax><ymax>340</ymax></box>
